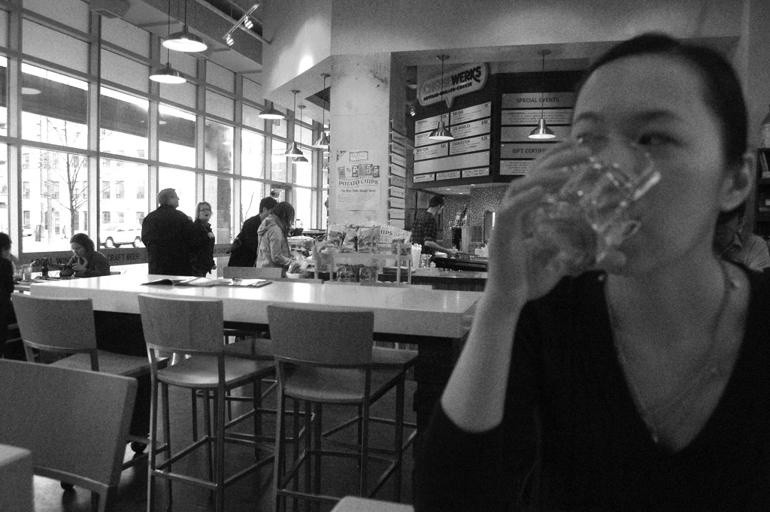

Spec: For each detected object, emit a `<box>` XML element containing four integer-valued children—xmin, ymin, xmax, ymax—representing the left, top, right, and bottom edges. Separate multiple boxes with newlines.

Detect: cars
<box><xmin>22</xmin><ymin>225</ymin><xmax>33</xmax><ymax>237</ymax></box>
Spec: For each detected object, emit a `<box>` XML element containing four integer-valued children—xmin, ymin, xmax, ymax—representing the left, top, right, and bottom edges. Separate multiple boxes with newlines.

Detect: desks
<box><xmin>0</xmin><ymin>444</ymin><xmax>34</xmax><ymax>512</ymax></box>
<box><xmin>29</xmin><ymin>273</ymin><xmax>487</xmax><ymax>510</ymax></box>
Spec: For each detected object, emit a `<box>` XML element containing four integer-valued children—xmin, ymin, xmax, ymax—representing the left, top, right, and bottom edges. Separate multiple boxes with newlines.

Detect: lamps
<box><xmin>218</xmin><ymin>0</ymin><xmax>260</xmax><ymax>48</ymax></box>
<box><xmin>258</xmin><ymin>99</ymin><xmax>286</xmax><ymax>120</ymax></box>
<box><xmin>162</xmin><ymin>0</ymin><xmax>208</xmax><ymax>56</ymax></box>
<box><xmin>293</xmin><ymin>99</ymin><xmax>312</xmax><ymax>164</ymax></box>
<box><xmin>148</xmin><ymin>1</ymin><xmax>193</xmax><ymax>88</ymax></box>
<box><xmin>284</xmin><ymin>88</ymin><xmax>307</xmax><ymax>160</ymax></box>
<box><xmin>315</xmin><ymin>71</ymin><xmax>332</xmax><ymax>151</ymax></box>
<box><xmin>526</xmin><ymin>48</ymin><xmax>558</xmax><ymax>141</ymax></box>
<box><xmin>428</xmin><ymin>53</ymin><xmax>456</xmax><ymax>140</ymax></box>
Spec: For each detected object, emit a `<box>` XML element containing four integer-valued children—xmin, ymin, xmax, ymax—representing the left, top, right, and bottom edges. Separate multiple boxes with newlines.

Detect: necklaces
<box><xmin>606</xmin><ymin>251</ymin><xmax>732</xmax><ymax>447</ymax></box>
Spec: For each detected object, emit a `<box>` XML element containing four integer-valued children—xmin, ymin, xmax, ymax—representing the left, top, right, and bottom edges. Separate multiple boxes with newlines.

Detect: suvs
<box><xmin>99</xmin><ymin>219</ymin><xmax>144</xmax><ymax>249</ymax></box>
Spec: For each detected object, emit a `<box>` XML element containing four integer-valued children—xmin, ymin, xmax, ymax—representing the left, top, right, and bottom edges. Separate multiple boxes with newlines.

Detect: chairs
<box><xmin>191</xmin><ymin>266</ymin><xmax>427</xmax><ymax>462</ymax></box>
<box><xmin>0</xmin><ymin>265</ymin><xmax>117</xmax><ymax>358</ymax></box>
<box><xmin>267</xmin><ymin>301</ymin><xmax>406</xmax><ymax>512</ymax></box>
<box><xmin>11</xmin><ymin>292</ymin><xmax>169</xmax><ymax>490</ymax></box>
<box><xmin>0</xmin><ymin>358</ymin><xmax>137</xmax><ymax>512</ymax></box>
<box><xmin>137</xmin><ymin>294</ymin><xmax>277</xmax><ymax>510</ymax></box>
<box><xmin>224</xmin><ymin>234</ymin><xmax>485</xmax><ymax>290</ymax></box>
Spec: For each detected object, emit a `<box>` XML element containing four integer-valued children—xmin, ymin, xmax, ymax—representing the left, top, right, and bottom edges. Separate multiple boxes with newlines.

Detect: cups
<box><xmin>523</xmin><ymin>131</ymin><xmax>663</xmax><ymax>274</ymax></box>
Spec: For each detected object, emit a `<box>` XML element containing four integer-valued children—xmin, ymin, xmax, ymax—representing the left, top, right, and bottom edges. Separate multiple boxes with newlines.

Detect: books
<box><xmin>759</xmin><ymin>151</ymin><xmax>769</xmax><ymax>172</ymax></box>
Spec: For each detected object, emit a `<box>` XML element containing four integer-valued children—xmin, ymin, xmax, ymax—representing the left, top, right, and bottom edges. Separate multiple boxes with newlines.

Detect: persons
<box><xmin>412</xmin><ymin>32</ymin><xmax>770</xmax><ymax>512</ymax></box>
<box><xmin>59</xmin><ymin>233</ymin><xmax>110</xmax><ymax>278</ymax></box>
<box><xmin>714</xmin><ymin>200</ymin><xmax>769</xmax><ymax>273</ymax></box>
<box><xmin>0</xmin><ymin>232</ymin><xmax>15</xmax><ymax>321</ymax></box>
<box><xmin>141</xmin><ymin>188</ymin><xmax>295</xmax><ymax>277</ymax></box>
<box><xmin>410</xmin><ymin>196</ymin><xmax>458</xmax><ymax>261</ymax></box>
